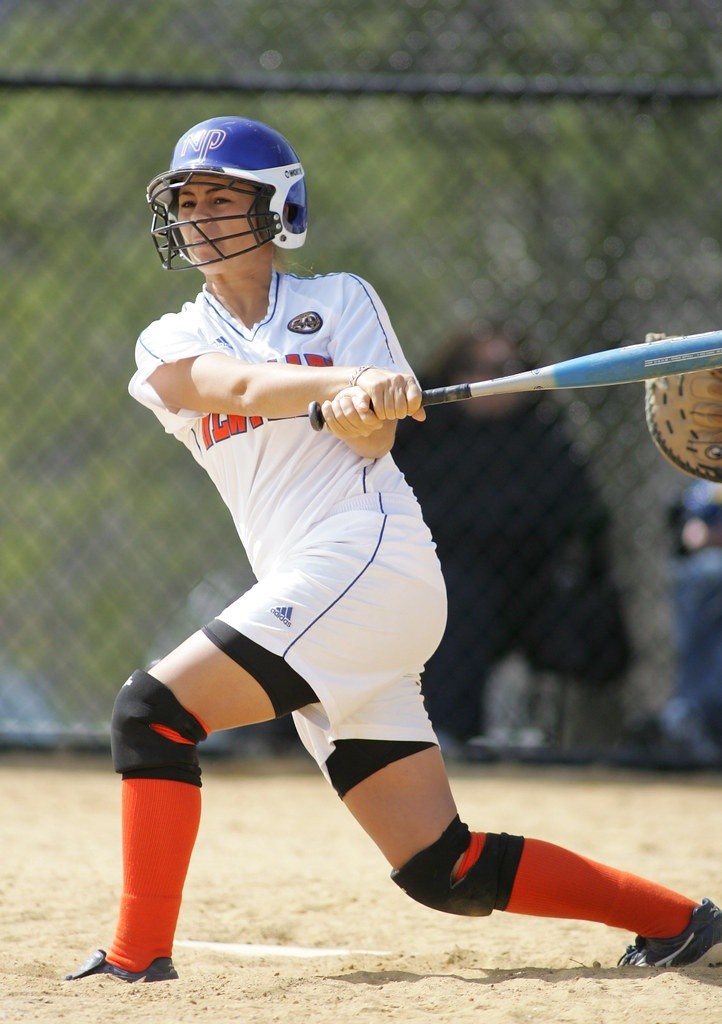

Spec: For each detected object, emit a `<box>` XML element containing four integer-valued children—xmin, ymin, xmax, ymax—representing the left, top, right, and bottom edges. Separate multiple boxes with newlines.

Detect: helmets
<box><xmin>146</xmin><ymin>116</ymin><xmax>308</xmax><ymax>248</ymax></box>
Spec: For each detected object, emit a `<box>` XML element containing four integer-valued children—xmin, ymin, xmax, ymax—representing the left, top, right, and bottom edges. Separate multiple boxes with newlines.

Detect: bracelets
<box><xmin>350</xmin><ymin>366</ymin><xmax>376</xmax><ymax>387</ymax></box>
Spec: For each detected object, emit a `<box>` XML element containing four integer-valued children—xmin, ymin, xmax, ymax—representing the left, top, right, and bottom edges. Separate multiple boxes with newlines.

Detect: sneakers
<box><xmin>616</xmin><ymin>898</ymin><xmax>722</xmax><ymax>967</ymax></box>
<box><xmin>58</xmin><ymin>949</ymin><xmax>178</xmax><ymax>984</ymax></box>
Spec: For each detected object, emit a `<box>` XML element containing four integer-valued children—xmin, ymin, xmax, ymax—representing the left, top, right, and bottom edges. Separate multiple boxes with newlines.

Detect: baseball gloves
<box><xmin>645</xmin><ymin>330</ymin><xmax>722</xmax><ymax>485</ymax></box>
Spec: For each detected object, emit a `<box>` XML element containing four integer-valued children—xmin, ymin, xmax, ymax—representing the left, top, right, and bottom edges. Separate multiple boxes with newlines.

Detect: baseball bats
<box><xmin>305</xmin><ymin>330</ymin><xmax>722</xmax><ymax>430</ymax></box>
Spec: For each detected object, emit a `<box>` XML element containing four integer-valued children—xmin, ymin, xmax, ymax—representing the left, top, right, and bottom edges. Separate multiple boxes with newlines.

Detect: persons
<box><xmin>387</xmin><ymin>316</ymin><xmax>722</xmax><ymax>777</ymax></box>
<box><xmin>64</xmin><ymin>116</ymin><xmax>722</xmax><ymax>983</ymax></box>
